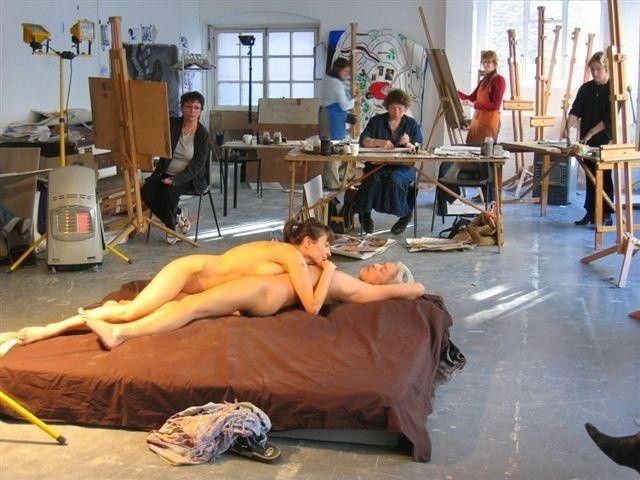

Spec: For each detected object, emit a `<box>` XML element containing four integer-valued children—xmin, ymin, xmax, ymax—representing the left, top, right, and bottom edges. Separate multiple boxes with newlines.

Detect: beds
<box><xmin>0</xmin><ymin>276</ymin><xmax>461</xmax><ymax>463</ymax></box>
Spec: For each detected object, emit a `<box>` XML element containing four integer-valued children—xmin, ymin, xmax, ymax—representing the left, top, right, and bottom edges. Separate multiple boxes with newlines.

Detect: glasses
<box><xmin>182</xmin><ymin>105</ymin><xmax>205</xmax><ymax>112</ymax></box>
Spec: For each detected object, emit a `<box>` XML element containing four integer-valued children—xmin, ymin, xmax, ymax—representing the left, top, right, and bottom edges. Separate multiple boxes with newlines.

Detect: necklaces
<box><xmin>180</xmin><ymin>120</ymin><xmax>196</xmax><ymax>138</ymax></box>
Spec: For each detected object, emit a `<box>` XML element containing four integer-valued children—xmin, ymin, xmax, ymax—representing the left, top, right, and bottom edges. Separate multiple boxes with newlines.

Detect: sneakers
<box><xmin>165</xmin><ymin>206</ymin><xmax>191</xmax><ymax>246</ymax></box>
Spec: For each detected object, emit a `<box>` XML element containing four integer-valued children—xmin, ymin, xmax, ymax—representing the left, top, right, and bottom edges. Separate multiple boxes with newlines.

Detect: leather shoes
<box><xmin>391</xmin><ymin>212</ymin><xmax>413</xmax><ymax>235</ymax></box>
<box><xmin>361</xmin><ymin>220</ymin><xmax>374</xmax><ymax>234</ymax></box>
<box><xmin>471</xmin><ymin>194</ymin><xmax>482</xmax><ymax>203</ymax></box>
<box><xmin>584</xmin><ymin>422</ymin><xmax>640</xmax><ymax>474</ymax></box>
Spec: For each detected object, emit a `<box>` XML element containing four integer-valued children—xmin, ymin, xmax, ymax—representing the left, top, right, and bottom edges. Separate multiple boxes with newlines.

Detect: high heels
<box><xmin>574</xmin><ymin>213</ymin><xmax>594</xmax><ymax>226</ymax></box>
<box><xmin>590</xmin><ymin>218</ymin><xmax>612</xmax><ymax>231</ymax></box>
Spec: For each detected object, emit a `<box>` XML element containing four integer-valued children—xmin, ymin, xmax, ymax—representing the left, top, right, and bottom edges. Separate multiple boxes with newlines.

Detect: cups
<box><xmin>350</xmin><ymin>143</ymin><xmax>359</xmax><ymax>157</ymax></box>
<box><xmin>244</xmin><ymin>135</ymin><xmax>252</xmax><ymax>144</ymax></box>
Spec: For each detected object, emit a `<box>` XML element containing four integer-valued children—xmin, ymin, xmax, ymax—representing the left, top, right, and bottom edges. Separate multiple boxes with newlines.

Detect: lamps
<box><xmin>20</xmin><ymin>20</ymin><xmax>97</xmax><ymax>169</ymax></box>
<box><xmin>238</xmin><ymin>34</ymin><xmax>255</xmax><ymax>126</ymax></box>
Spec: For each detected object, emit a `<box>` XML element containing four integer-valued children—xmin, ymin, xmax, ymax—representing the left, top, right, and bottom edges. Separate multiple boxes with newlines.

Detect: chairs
<box><xmin>146</xmin><ymin>149</ymin><xmax>222</xmax><ymax>245</ymax></box>
<box><xmin>219</xmin><ymin>127</ymin><xmax>262</xmax><ymax>199</ymax></box>
<box><xmin>431</xmin><ymin>163</ymin><xmax>495</xmax><ymax>233</ymax></box>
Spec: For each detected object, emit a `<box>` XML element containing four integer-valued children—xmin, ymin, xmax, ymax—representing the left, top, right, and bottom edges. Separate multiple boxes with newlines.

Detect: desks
<box><xmin>281</xmin><ymin>146</ymin><xmax>508</xmax><ymax>255</ymax></box>
<box><xmin>502</xmin><ymin>139</ymin><xmax>639</xmax><ymax>251</ymax></box>
<box><xmin>219</xmin><ymin>140</ymin><xmax>308</xmax><ymax>216</ymax></box>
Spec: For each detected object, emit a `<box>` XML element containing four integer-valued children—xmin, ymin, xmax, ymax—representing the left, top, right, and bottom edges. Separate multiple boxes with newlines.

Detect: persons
<box><xmin>357</xmin><ymin>90</ymin><xmax>422</xmax><ymax>235</ymax></box>
<box><xmin>77</xmin><ymin>261</ymin><xmax>423</xmax><ymax>350</ymax></box>
<box><xmin>456</xmin><ymin>51</ymin><xmax>505</xmax><ymax>204</ymax></box>
<box><xmin>140</xmin><ymin>91</ymin><xmax>211</xmax><ymax>246</ymax></box>
<box><xmin>559</xmin><ymin>52</ymin><xmax>622</xmax><ymax>230</ymax></box>
<box><xmin>16</xmin><ymin>217</ymin><xmax>335</xmax><ymax>347</ymax></box>
<box><xmin>318</xmin><ymin>58</ymin><xmax>363</xmax><ymax>191</ymax></box>
